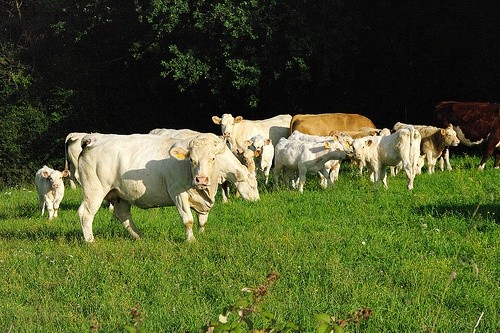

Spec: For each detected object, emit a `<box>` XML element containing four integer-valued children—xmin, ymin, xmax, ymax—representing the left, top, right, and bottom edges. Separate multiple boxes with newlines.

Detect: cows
<box><xmin>393</xmin><ymin>122</ymin><xmax>460</xmax><ymax>174</ymax></box>
<box><xmin>433</xmin><ymin>101</ymin><xmax>500</xmax><ymax>172</ymax></box>
<box><xmin>35</xmin><ymin>165</ymin><xmax>70</xmax><ymax>220</ymax></box>
<box><xmin>347</xmin><ymin>125</ymin><xmax>421</xmax><ymax>190</ymax></box>
<box><xmin>290</xmin><ymin>113</ymin><xmax>375</xmax><ymax>169</ymax></box>
<box><xmin>77</xmin><ymin>133</ymin><xmax>226</xmax><ymax>243</ymax></box>
<box><xmin>273</xmin><ymin>137</ymin><xmax>346</xmax><ymax>194</ymax></box>
<box><xmin>212</xmin><ymin>114</ymin><xmax>292</xmax><ymax>165</ymax></box>
<box><xmin>65</xmin><ymin>127</ymin><xmax>426</xmax><ymax>211</ymax></box>
